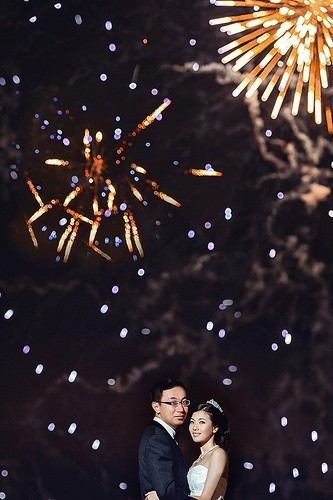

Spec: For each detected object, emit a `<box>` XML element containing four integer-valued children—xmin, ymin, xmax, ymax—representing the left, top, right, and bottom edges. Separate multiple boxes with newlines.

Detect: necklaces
<box><xmin>193</xmin><ymin>445</ymin><xmax>221</xmax><ymax>466</ymax></box>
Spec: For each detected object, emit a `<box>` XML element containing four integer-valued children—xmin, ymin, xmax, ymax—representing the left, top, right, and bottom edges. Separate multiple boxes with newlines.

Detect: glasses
<box><xmin>159</xmin><ymin>399</ymin><xmax>190</xmax><ymax>408</ymax></box>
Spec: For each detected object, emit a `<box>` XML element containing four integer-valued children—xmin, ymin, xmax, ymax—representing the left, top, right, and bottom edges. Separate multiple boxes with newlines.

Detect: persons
<box><xmin>144</xmin><ymin>400</ymin><xmax>230</xmax><ymax>500</ymax></box>
<box><xmin>138</xmin><ymin>380</ymin><xmax>198</xmax><ymax>500</ymax></box>
